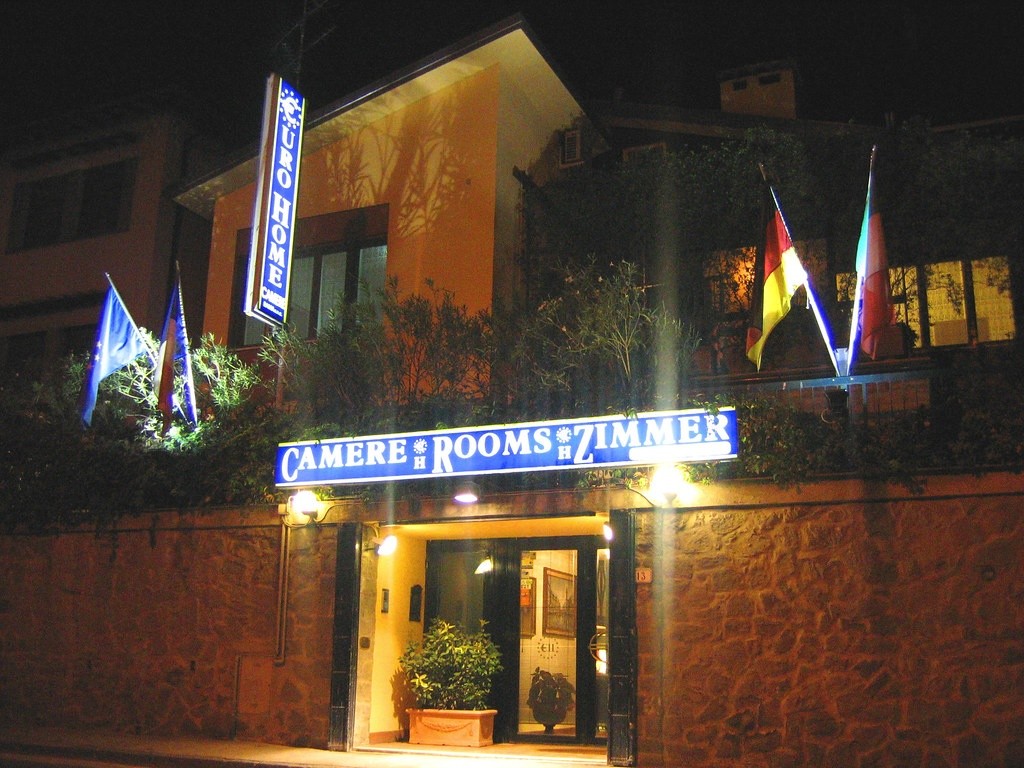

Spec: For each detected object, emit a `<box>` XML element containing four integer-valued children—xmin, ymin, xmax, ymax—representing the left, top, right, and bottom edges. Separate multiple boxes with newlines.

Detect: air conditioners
<box><xmin>562</xmin><ymin>128</ymin><xmax>591</xmax><ymax>163</ymax></box>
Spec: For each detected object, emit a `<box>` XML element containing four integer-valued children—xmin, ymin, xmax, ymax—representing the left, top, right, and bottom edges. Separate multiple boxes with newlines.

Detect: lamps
<box><xmin>454</xmin><ymin>478</ymin><xmax>478</xmax><ymax>503</ymax></box>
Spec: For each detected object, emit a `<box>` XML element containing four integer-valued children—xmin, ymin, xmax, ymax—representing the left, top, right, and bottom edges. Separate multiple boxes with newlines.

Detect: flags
<box><xmin>78</xmin><ymin>284</ymin><xmax>145</xmax><ymax>431</ymax></box>
<box><xmin>153</xmin><ymin>268</ymin><xmax>198</xmax><ymax>438</ymax></box>
<box><xmin>746</xmin><ymin>181</ymin><xmax>836</xmax><ymax>373</ymax></box>
<box><xmin>853</xmin><ymin>163</ymin><xmax>893</xmax><ymax>362</ymax></box>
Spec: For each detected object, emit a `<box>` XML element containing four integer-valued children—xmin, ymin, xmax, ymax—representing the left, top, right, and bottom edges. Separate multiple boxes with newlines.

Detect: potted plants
<box><xmin>397</xmin><ymin>616</ymin><xmax>503</xmax><ymax>748</ymax></box>
<box><xmin>530</xmin><ymin>667</ymin><xmax>585</xmax><ymax>741</ymax></box>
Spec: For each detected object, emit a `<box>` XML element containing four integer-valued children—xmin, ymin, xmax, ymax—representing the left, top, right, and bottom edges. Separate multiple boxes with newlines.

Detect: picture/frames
<box><xmin>521</xmin><ymin>577</ymin><xmax>536</xmax><ymax>637</ymax></box>
<box><xmin>542</xmin><ymin>568</ymin><xmax>576</xmax><ymax>638</ymax></box>
